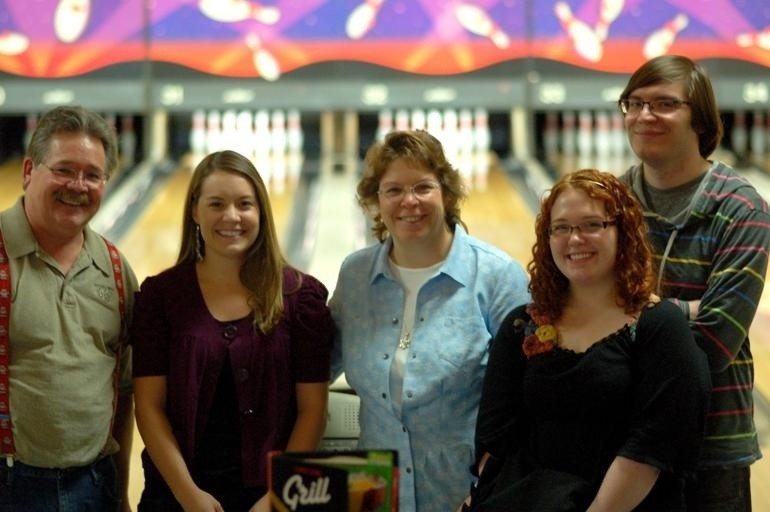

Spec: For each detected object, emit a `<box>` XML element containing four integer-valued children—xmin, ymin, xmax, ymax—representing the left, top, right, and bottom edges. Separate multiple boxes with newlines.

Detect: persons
<box><xmin>1</xmin><ymin>104</ymin><xmax>139</xmax><ymax>512</ymax></box>
<box><xmin>316</xmin><ymin>127</ymin><xmax>537</xmax><ymax>511</ymax></box>
<box><xmin>607</xmin><ymin>51</ymin><xmax>769</xmax><ymax>511</ymax></box>
<box><xmin>464</xmin><ymin>167</ymin><xmax>711</xmax><ymax>511</ymax></box>
<box><xmin>129</xmin><ymin>149</ymin><xmax>336</xmax><ymax>511</ymax></box>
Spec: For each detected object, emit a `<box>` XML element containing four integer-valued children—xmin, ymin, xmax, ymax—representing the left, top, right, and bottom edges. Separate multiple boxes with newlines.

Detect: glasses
<box><xmin>375</xmin><ymin>178</ymin><xmax>441</xmax><ymax>208</ymax></box>
<box><xmin>543</xmin><ymin>213</ymin><xmax>614</xmax><ymax>244</ymax></box>
<box><xmin>616</xmin><ymin>95</ymin><xmax>685</xmax><ymax>118</ymax></box>
<box><xmin>40</xmin><ymin>162</ymin><xmax>111</xmax><ymax>191</ymax></box>
